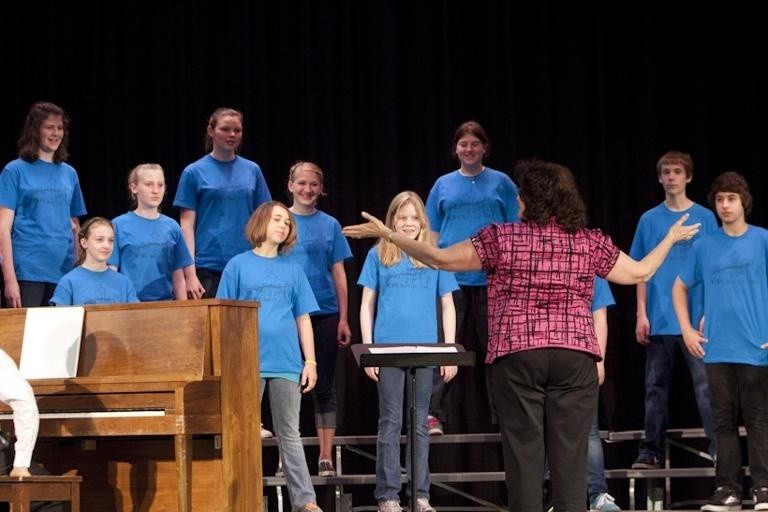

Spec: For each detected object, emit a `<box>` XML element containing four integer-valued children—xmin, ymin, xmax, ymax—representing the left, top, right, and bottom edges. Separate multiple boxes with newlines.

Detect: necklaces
<box><xmin>458</xmin><ymin>164</ymin><xmax>486</xmax><ymax>185</ymax></box>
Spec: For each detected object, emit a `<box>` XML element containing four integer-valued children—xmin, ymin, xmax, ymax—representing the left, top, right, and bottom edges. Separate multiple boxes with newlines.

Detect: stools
<box><xmin>0</xmin><ymin>475</ymin><xmax>83</xmax><ymax>512</ymax></box>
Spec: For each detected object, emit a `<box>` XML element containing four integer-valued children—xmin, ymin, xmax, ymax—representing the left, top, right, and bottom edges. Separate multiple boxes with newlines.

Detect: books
<box><xmin>19</xmin><ymin>307</ymin><xmax>85</xmax><ymax>378</ymax></box>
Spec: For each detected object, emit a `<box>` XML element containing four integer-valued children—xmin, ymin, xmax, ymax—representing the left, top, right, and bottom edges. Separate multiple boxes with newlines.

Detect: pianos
<box><xmin>1</xmin><ymin>299</ymin><xmax>264</xmax><ymax>510</ymax></box>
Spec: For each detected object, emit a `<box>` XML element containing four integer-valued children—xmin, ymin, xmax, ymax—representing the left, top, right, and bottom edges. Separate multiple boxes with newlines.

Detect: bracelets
<box><xmin>304</xmin><ymin>358</ymin><xmax>318</xmax><ymax>365</ymax></box>
<box><xmin>386</xmin><ymin>230</ymin><xmax>394</xmax><ymax>242</ymax></box>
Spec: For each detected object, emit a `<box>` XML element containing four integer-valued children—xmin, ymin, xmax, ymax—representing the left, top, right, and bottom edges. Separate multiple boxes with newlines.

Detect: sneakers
<box><xmin>377</xmin><ymin>500</ymin><xmax>402</xmax><ymax>511</ymax></box>
<box><xmin>589</xmin><ymin>492</ymin><xmax>621</xmax><ymax>512</ymax></box>
<box><xmin>260</xmin><ymin>423</ymin><xmax>273</xmax><ymax>438</ymax></box>
<box><xmin>701</xmin><ymin>486</ymin><xmax>742</xmax><ymax>512</ymax></box>
<box><xmin>318</xmin><ymin>459</ymin><xmax>334</xmax><ymax>477</ymax></box>
<box><xmin>275</xmin><ymin>465</ymin><xmax>285</xmax><ymax>477</ymax></box>
<box><xmin>632</xmin><ymin>449</ymin><xmax>664</xmax><ymax>468</ymax></box>
<box><xmin>752</xmin><ymin>486</ymin><xmax>768</xmax><ymax>511</ymax></box>
<box><xmin>407</xmin><ymin>497</ymin><xmax>436</xmax><ymax>512</ymax></box>
<box><xmin>300</xmin><ymin>502</ymin><xmax>322</xmax><ymax>512</ymax></box>
<box><xmin>428</xmin><ymin>415</ymin><xmax>443</xmax><ymax>435</ymax></box>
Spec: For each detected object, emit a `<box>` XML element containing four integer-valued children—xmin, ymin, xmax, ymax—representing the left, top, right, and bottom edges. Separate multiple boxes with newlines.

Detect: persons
<box><xmin>173</xmin><ymin>106</ymin><xmax>274</xmax><ymax>298</ymax></box>
<box><xmin>670</xmin><ymin>170</ymin><xmax>768</xmax><ymax>512</ymax></box>
<box><xmin>629</xmin><ymin>150</ymin><xmax>722</xmax><ymax>469</ymax></box>
<box><xmin>48</xmin><ymin>216</ymin><xmax>140</xmax><ymax>307</ymax></box>
<box><xmin>0</xmin><ymin>104</ymin><xmax>89</xmax><ymax>307</ymax></box>
<box><xmin>425</xmin><ymin>122</ymin><xmax>522</xmax><ymax>437</ymax></box>
<box><xmin>106</xmin><ymin>162</ymin><xmax>193</xmax><ymax>301</ymax></box>
<box><xmin>216</xmin><ymin>202</ymin><xmax>324</xmax><ymax>511</ymax></box>
<box><xmin>356</xmin><ymin>191</ymin><xmax>460</xmax><ymax>511</ymax></box>
<box><xmin>587</xmin><ymin>274</ymin><xmax>622</xmax><ymax>511</ymax></box>
<box><xmin>342</xmin><ymin>162</ymin><xmax>703</xmax><ymax>512</ymax></box>
<box><xmin>273</xmin><ymin>162</ymin><xmax>352</xmax><ymax>478</ymax></box>
<box><xmin>0</xmin><ymin>348</ymin><xmax>65</xmax><ymax>511</ymax></box>
